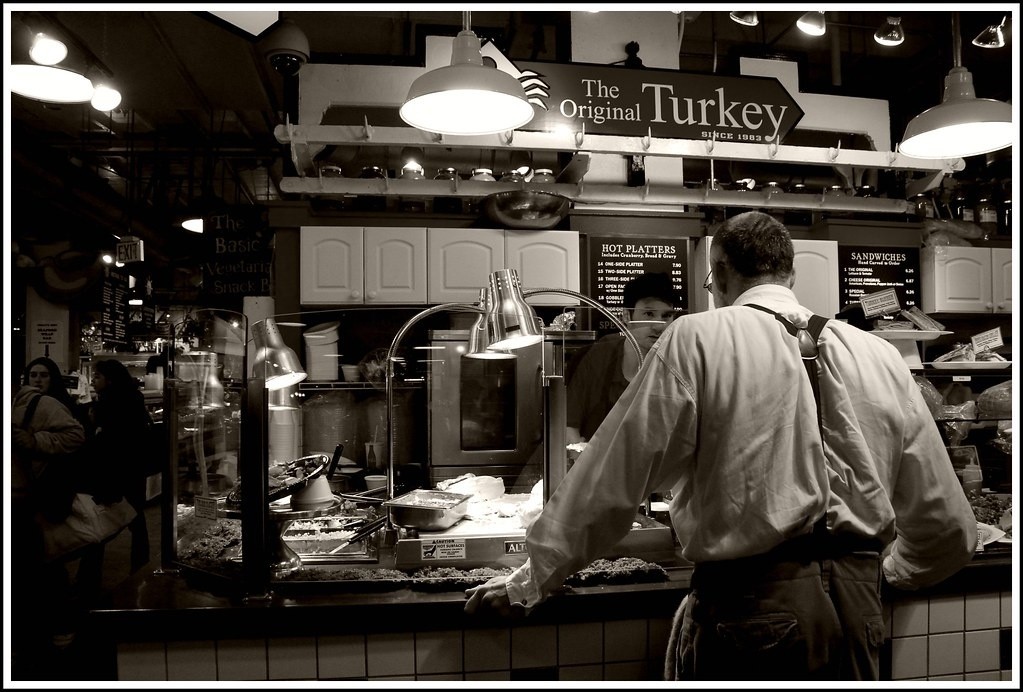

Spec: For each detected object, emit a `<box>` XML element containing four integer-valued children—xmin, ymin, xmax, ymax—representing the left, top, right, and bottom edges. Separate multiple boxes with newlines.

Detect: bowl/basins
<box><xmin>342</xmin><ymin>365</ymin><xmax>360</xmax><ymax>381</ymax></box>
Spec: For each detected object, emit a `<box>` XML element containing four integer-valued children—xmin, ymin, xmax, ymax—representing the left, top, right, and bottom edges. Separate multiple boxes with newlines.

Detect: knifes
<box><xmin>329</xmin><ymin>522</ymin><xmax>385</xmax><ymax>555</ymax></box>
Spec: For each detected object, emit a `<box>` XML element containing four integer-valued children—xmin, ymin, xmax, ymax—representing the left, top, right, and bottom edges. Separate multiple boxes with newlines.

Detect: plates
<box><xmin>868</xmin><ymin>330</ymin><xmax>954</xmax><ymax>341</ymax></box>
<box><xmin>303</xmin><ymin>320</ymin><xmax>340</xmax><ymax>381</ymax></box>
<box><xmin>922</xmin><ymin>361</ymin><xmax>1012</xmax><ymax>369</ymax></box>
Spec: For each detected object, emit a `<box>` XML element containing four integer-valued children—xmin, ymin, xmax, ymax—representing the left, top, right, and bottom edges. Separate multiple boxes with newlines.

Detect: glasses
<box><xmin>704</xmin><ymin>266</ymin><xmax>717</xmax><ymax>293</ymax></box>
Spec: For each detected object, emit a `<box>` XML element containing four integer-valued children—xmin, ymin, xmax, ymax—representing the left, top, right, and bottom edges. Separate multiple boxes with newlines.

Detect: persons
<box><xmin>87</xmin><ymin>359</ymin><xmax>155</xmax><ymax>573</ymax></box>
<box><xmin>23</xmin><ymin>358</ymin><xmax>77</xmax><ymax>413</ymax></box>
<box><xmin>462</xmin><ymin>213</ymin><xmax>975</xmax><ymax>680</ymax></box>
<box><xmin>565</xmin><ymin>271</ymin><xmax>674</xmax><ymax>447</ymax></box>
<box><xmin>12</xmin><ymin>371</ymin><xmax>84</xmax><ymax>563</ymax></box>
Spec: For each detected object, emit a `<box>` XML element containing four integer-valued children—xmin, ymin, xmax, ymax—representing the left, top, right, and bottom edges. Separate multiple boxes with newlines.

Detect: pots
<box><xmin>479</xmin><ymin>190</ymin><xmax>575</xmax><ymax>231</ymax></box>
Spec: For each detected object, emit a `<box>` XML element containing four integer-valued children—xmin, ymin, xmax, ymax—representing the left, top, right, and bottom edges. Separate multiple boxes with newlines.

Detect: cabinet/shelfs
<box><xmin>300</xmin><ymin>224</ymin><xmax>580</xmax><ymax>308</ymax></box>
<box><xmin>692</xmin><ymin>236</ymin><xmax>839</xmax><ymax>317</ymax></box>
<box><xmin>920</xmin><ymin>244</ymin><xmax>1011</xmax><ymax>313</ymax></box>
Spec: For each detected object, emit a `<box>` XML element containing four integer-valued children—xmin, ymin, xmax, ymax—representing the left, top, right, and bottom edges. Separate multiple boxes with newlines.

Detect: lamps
<box><xmin>486</xmin><ymin>268</ymin><xmax>643</xmax><ymax>373</ymax></box>
<box><xmin>796</xmin><ymin>10</ymin><xmax>825</xmax><ymax>34</ymax></box>
<box><xmin>250</xmin><ymin>319</ymin><xmax>307</xmax><ymax>391</ymax></box>
<box><xmin>898</xmin><ymin>12</ymin><xmax>1013</xmax><ymax>160</ymax></box>
<box><xmin>874</xmin><ymin>14</ymin><xmax>904</xmax><ymax>44</ymax></box>
<box><xmin>974</xmin><ymin>16</ymin><xmax>1005</xmax><ymax>48</ymax></box>
<box><xmin>730</xmin><ymin>10</ymin><xmax>757</xmax><ymax>25</ymax></box>
<box><xmin>401</xmin><ymin>11</ymin><xmax>536</xmax><ymax>137</ymax></box>
<box><xmin>384</xmin><ymin>287</ymin><xmax>519</xmax><ymax>546</ymax></box>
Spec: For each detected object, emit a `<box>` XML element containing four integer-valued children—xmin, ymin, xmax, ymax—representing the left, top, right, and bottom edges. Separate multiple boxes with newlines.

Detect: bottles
<box><xmin>674</xmin><ymin>179</ymin><xmax>1012</xmax><ymax>238</ymax></box>
<box><xmin>368</xmin><ymin>445</ymin><xmax>376</xmax><ymax>468</ymax></box>
<box><xmin>318</xmin><ymin>160</ymin><xmax>556</xmax><ymax>212</ymax></box>
<box><xmin>963</xmin><ymin>457</ymin><xmax>982</xmax><ymax>496</ymax></box>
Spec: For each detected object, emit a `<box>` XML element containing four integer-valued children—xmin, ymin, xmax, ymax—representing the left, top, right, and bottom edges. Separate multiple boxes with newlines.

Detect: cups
<box><xmin>364</xmin><ymin>475</ymin><xmax>387</xmax><ymax>490</ymax></box>
<box><xmin>363</xmin><ymin>441</ymin><xmax>384</xmax><ymax>468</ymax></box>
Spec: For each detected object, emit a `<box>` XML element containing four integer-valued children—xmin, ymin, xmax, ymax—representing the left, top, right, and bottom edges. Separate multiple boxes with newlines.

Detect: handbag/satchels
<box><xmin>17</xmin><ymin>394</ymin><xmax>137</xmax><ymax>559</ymax></box>
<box><xmin>134</xmin><ymin>404</ymin><xmax>168</xmax><ymax>478</ymax></box>
